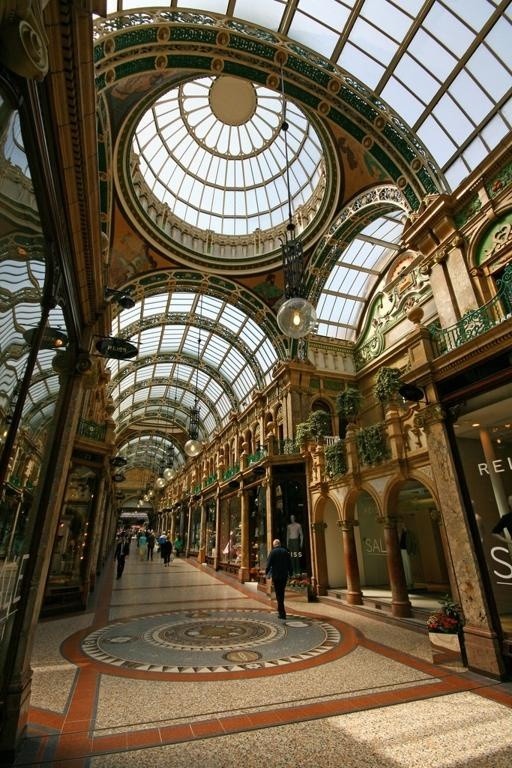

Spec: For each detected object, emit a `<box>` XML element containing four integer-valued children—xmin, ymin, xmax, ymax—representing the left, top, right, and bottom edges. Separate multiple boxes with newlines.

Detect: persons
<box><xmin>265</xmin><ymin>537</ymin><xmax>292</xmax><ymax>619</ymax></box>
<box><xmin>491</xmin><ymin>492</ymin><xmax>512</xmax><ymax>545</ymax></box>
<box><xmin>398</xmin><ymin>523</ymin><xmax>415</xmax><ymax>591</ymax></box>
<box><xmin>114</xmin><ymin>524</ymin><xmax>184</xmax><ymax>580</ymax></box>
<box><xmin>286</xmin><ymin>515</ymin><xmax>306</xmax><ymax>574</ymax></box>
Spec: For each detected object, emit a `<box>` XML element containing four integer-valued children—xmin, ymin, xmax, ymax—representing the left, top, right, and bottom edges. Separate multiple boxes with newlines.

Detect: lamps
<box><xmin>277</xmin><ymin>66</ymin><xmax>318</xmax><ymax>340</ymax></box>
<box><xmin>138</xmin><ymin>286</ymin><xmax>205</xmax><ymax>505</ymax></box>
<box><xmin>103</xmin><ymin>286</ymin><xmax>137</xmax><ymax>309</ymax></box>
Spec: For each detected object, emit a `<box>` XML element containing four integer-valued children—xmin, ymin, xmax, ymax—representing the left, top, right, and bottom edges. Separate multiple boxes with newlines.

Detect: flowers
<box><xmin>427</xmin><ymin>594</ymin><xmax>464</xmax><ymax>633</ymax></box>
